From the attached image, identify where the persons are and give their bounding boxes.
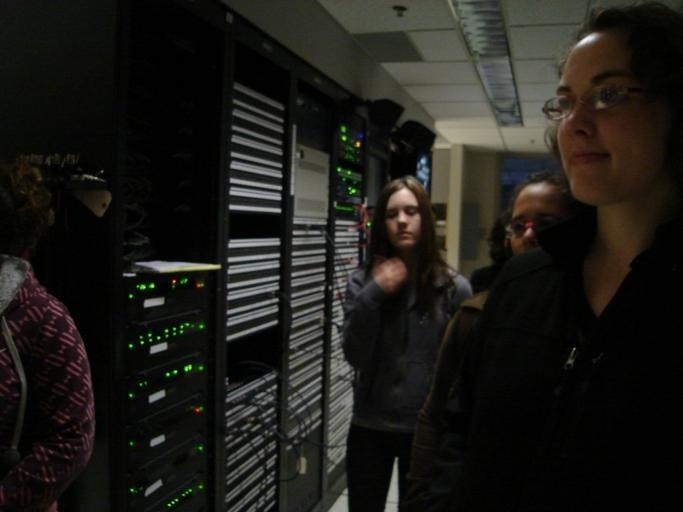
[0,153,96,510]
[470,205,513,294]
[427,0,682,512]
[341,174,474,512]
[399,168,569,511]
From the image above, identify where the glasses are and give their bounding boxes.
[496,220,545,240]
[541,82,646,122]
